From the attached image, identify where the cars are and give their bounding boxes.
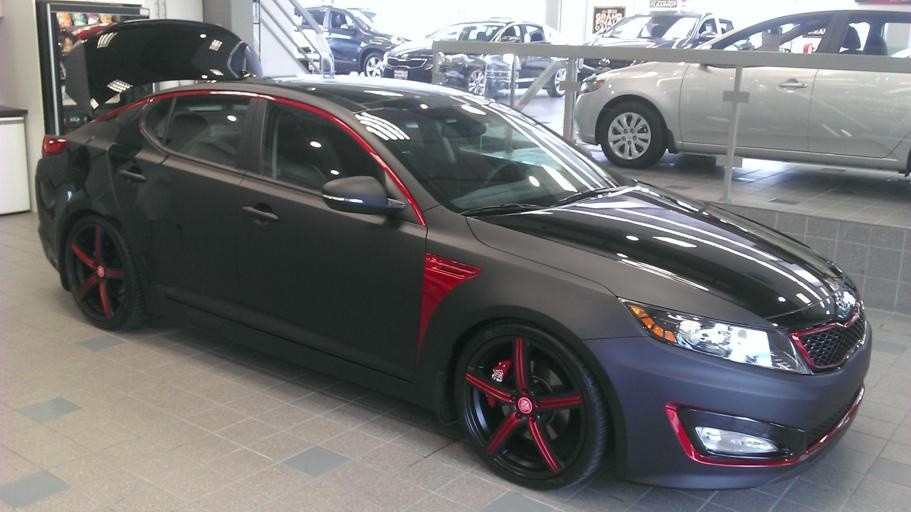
[574,3,911,177]
[575,8,734,84]
[378,18,570,98]
[30,19,874,494]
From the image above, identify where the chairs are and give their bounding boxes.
[168,113,236,167]
[840,27,862,56]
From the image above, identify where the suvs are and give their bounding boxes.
[292,5,413,78]
